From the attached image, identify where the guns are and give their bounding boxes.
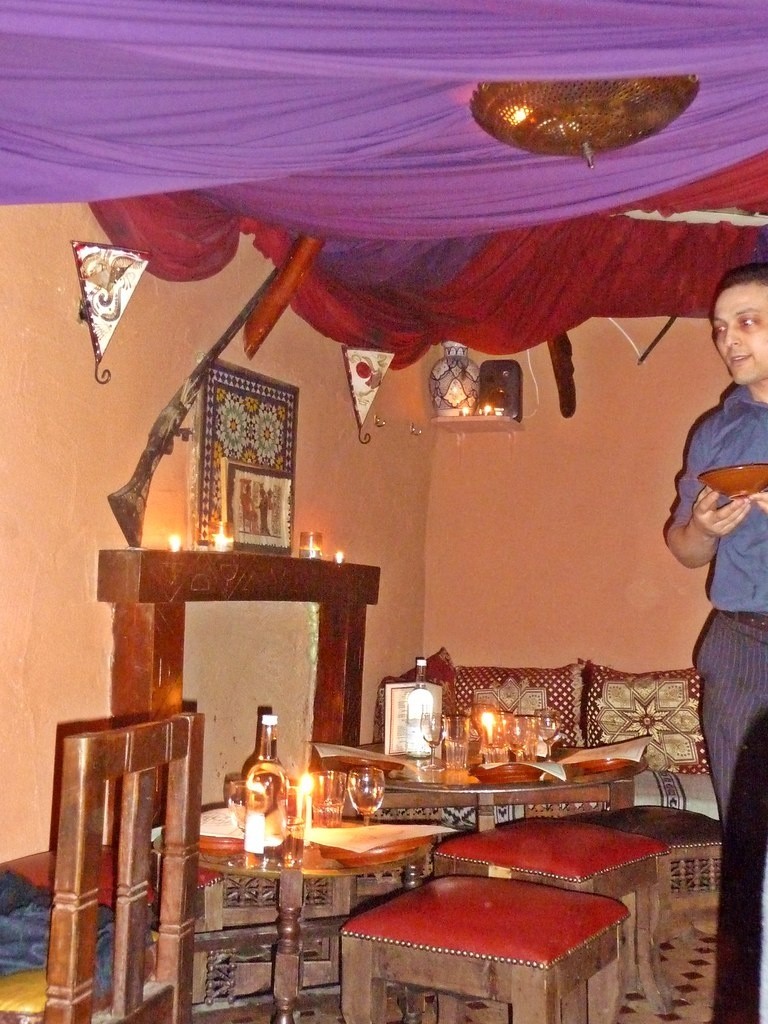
[109,261,280,548]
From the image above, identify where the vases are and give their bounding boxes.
[428,339,479,417]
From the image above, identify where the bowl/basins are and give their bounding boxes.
[198,835,243,858]
[320,835,433,869]
[698,463,768,500]
[336,756,405,780]
[469,765,545,783]
[569,756,637,775]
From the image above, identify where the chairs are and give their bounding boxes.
[1,711,206,1024]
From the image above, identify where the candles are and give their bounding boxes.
[298,771,315,847]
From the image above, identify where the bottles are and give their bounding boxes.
[407,658,436,758]
[429,341,480,417]
[244,714,289,855]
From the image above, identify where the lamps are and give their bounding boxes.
[466,74,701,169]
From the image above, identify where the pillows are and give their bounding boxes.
[450,658,589,752]
[583,661,710,776]
[372,645,457,741]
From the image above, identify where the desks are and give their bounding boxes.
[152,819,437,1024]
[334,747,646,836]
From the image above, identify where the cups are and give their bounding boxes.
[298,531,323,560]
[419,704,558,773]
[223,766,385,867]
[208,520,235,552]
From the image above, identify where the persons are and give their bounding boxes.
[663,260,768,826]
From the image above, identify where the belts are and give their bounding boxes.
[723,608,768,632]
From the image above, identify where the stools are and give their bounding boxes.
[433,817,671,1023]
[337,874,631,1024]
[585,804,724,996]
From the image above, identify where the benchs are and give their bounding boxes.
[375,772,723,909]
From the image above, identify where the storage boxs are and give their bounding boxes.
[384,680,444,755]
